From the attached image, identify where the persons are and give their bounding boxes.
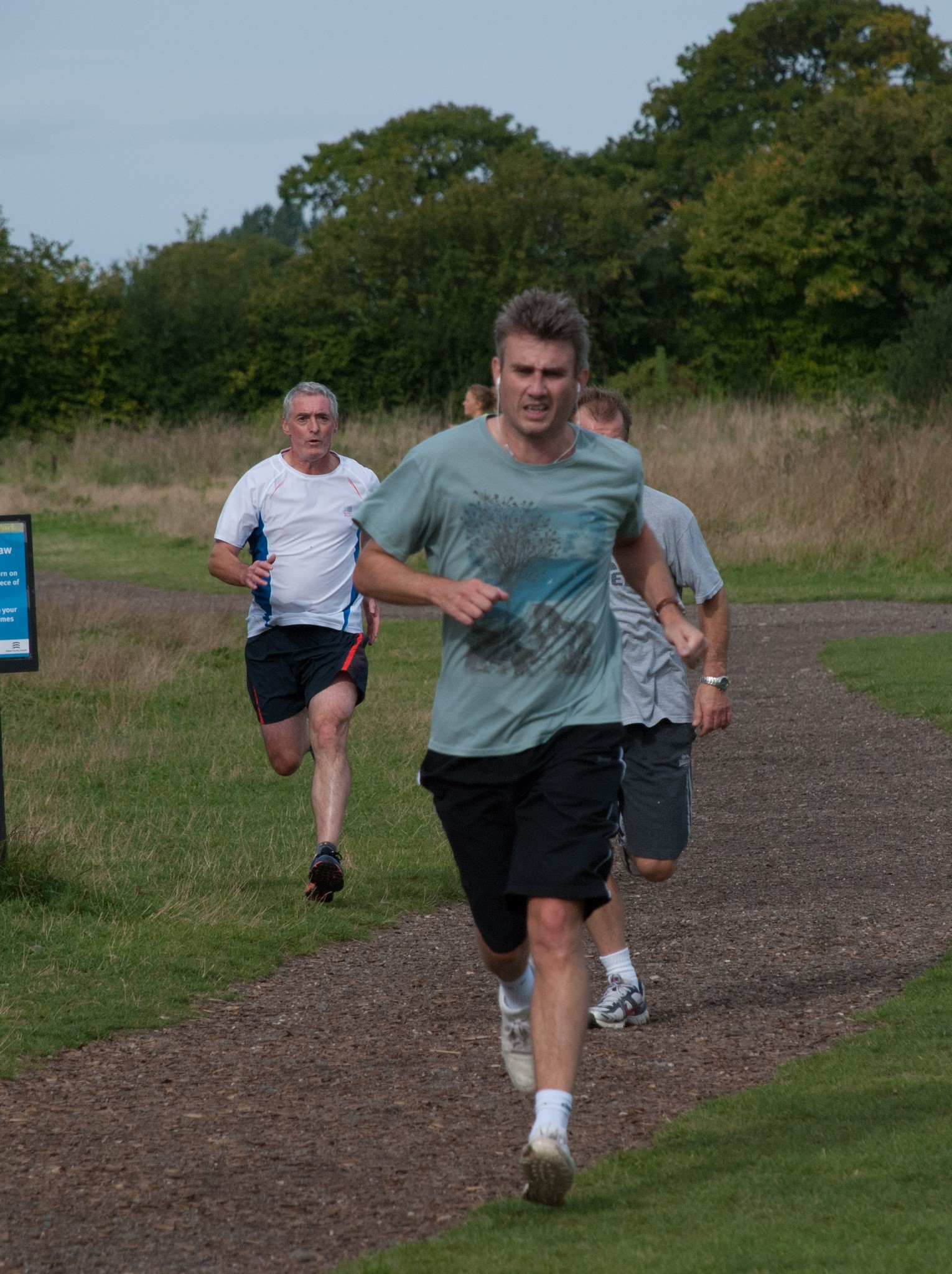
[569,391,732,1027]
[462,384,495,418]
[351,287,710,1207]
[207,382,381,901]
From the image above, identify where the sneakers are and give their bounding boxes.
[588,972,651,1028]
[304,846,345,903]
[519,1121,577,1208]
[499,957,538,1095]
[615,811,643,877]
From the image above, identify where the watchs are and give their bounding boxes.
[655,597,686,623]
[700,675,730,691]
